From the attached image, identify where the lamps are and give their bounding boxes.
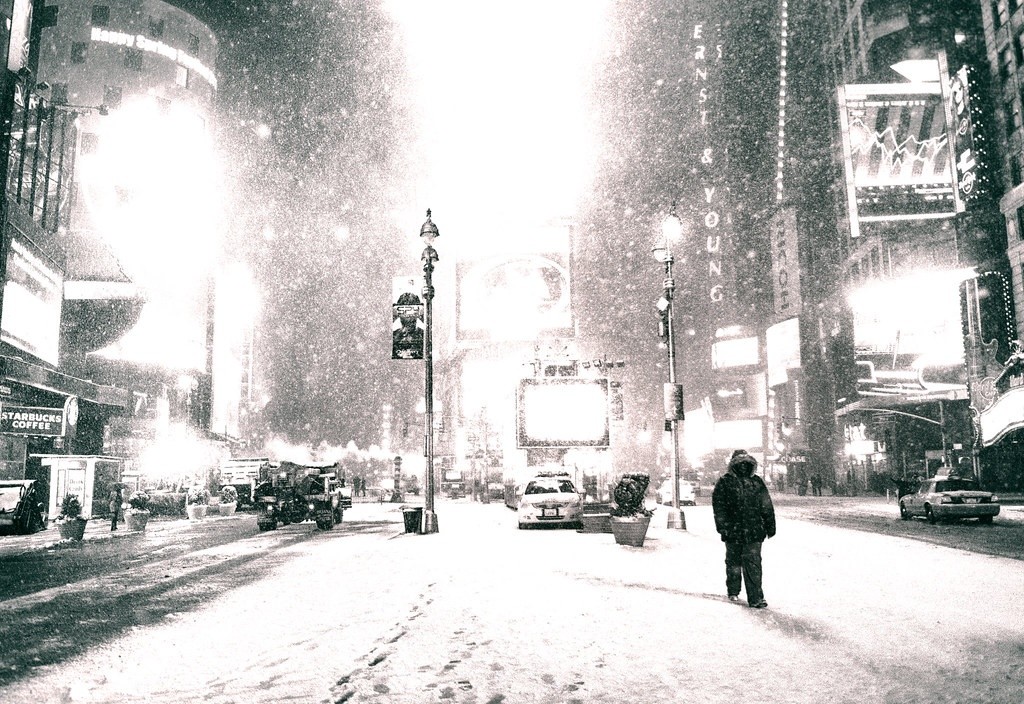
[37,81,50,91]
[18,66,32,77]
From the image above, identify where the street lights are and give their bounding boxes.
[846,401,949,467]
[649,198,693,530]
[418,208,440,532]
[779,412,849,496]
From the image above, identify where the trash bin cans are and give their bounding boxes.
[402,506,422,536]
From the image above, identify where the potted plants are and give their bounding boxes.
[607,473,657,546]
[218,486,238,516]
[52,492,88,540]
[187,490,210,520]
[124,491,150,530]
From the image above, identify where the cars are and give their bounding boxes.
[515,469,586,528]
[655,478,697,507]
[897,464,1002,524]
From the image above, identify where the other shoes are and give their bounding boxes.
[749,600,767,608]
[729,595,738,601]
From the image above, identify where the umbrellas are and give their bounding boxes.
[105,482,129,490]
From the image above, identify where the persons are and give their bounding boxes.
[353,475,366,498]
[109,496,118,531]
[116,489,125,522]
[810,473,823,497]
[712,449,777,610]
[341,478,345,488]
